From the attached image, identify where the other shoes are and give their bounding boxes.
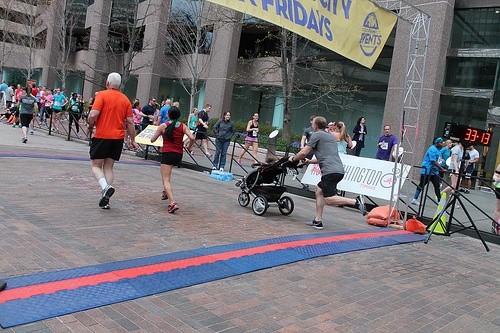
[464,190,470,194]
[30,131,34,135]
[204,153,213,158]
[220,168,224,171]
[237,162,243,166]
[458,188,465,192]
[188,150,194,155]
[0,118,22,128]
[21,137,27,143]
[450,203,460,208]
[411,200,422,206]
[212,166,216,170]
[492,225,500,235]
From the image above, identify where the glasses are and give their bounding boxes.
[152,100,155,102]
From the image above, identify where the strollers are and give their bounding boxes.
[235,156,308,216]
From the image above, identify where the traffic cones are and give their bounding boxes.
[426,191,448,234]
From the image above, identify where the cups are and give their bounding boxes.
[149,117,154,121]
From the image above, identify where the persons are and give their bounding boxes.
[0,80,100,134]
[87,73,138,209]
[352,118,368,156]
[212,111,235,171]
[488,164,500,235]
[186,108,198,155]
[297,114,353,154]
[289,116,366,229]
[150,107,194,214]
[124,96,180,155]
[17,87,39,143]
[376,125,398,161]
[238,112,261,168]
[190,104,214,157]
[413,137,480,210]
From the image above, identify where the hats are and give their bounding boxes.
[467,142,473,146]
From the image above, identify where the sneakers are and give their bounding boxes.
[306,218,324,229]
[167,202,179,213]
[101,198,110,209]
[98,184,115,207]
[355,194,367,216]
[161,190,168,200]
